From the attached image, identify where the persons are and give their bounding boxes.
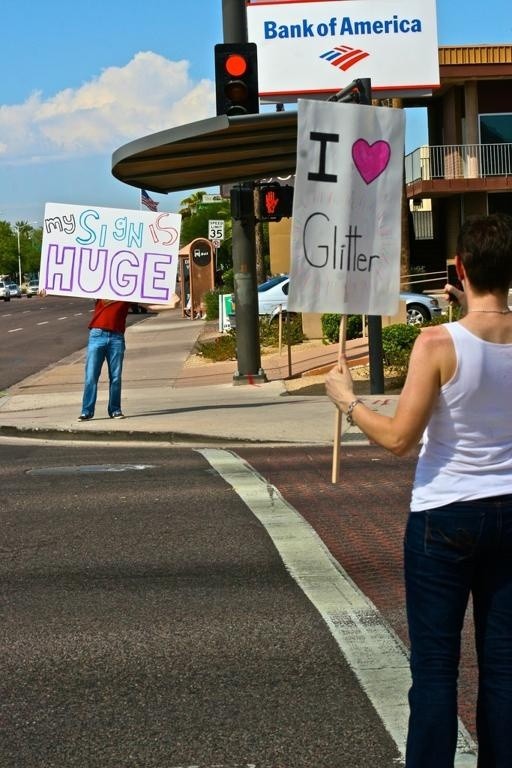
[324,215,512,768]
[38,289,180,422]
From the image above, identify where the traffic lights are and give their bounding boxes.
[259,186,293,217]
[229,186,255,220]
[213,42,260,117]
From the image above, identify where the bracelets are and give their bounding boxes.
[346,400,363,427]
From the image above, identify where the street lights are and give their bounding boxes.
[9,221,39,286]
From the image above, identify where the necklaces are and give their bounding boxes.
[474,307,510,315]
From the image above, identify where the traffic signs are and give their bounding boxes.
[208,219,224,241]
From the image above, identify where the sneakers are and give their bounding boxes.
[76,412,93,421]
[111,413,125,418]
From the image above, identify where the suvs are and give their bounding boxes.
[25,280,39,298]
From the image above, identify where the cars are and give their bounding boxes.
[0,280,22,302]
[256,271,444,331]
